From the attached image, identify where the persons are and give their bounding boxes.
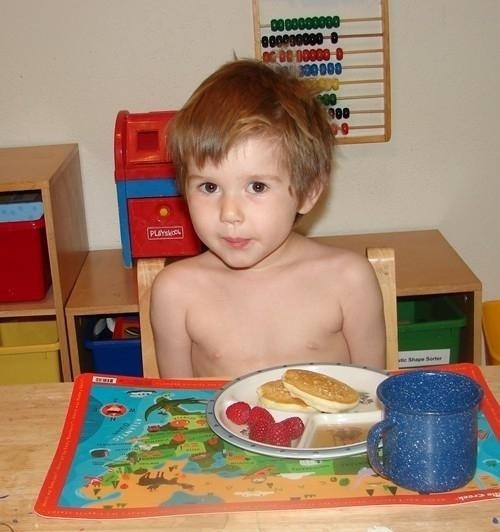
[150,60,387,378]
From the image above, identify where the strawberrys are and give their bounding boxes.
[279,417,305,440]
[249,420,271,443]
[248,406,275,427]
[225,401,251,425]
[267,423,291,447]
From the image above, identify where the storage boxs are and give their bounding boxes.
[86,315,142,379]
[1,200,50,305]
[1,318,65,384]
[397,296,468,369]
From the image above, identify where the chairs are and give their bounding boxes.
[138,248,399,379]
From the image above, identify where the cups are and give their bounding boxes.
[366,370,485,495]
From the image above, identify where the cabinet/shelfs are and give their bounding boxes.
[65,229,483,382]
[0,144,90,382]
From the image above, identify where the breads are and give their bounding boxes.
[259,369,361,413]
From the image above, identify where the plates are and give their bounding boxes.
[214,366,388,450]
[205,363,390,461]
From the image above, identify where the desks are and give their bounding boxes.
[0,366,499,532]
